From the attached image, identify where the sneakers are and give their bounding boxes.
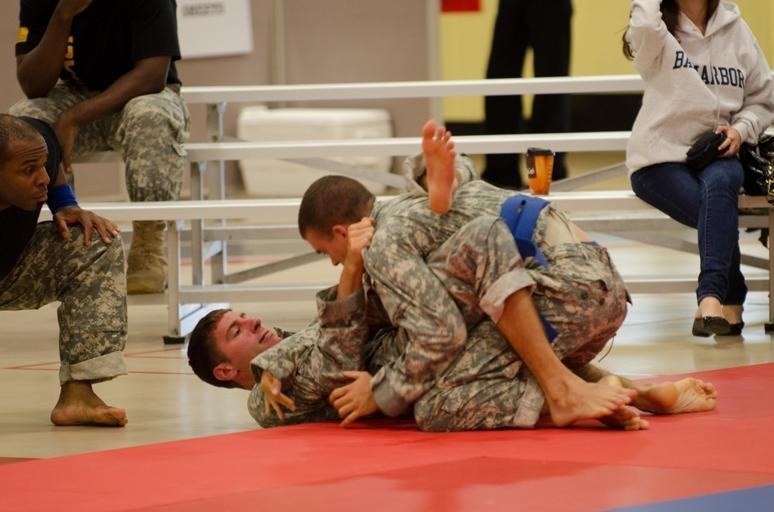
[126,229,169,294]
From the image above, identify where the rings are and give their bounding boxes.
[727,135,734,143]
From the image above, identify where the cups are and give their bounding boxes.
[524,147,555,197]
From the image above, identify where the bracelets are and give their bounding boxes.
[45,182,79,216]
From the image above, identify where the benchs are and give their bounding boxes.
[37,74,772,335]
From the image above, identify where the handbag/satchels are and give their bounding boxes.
[739,133,774,197]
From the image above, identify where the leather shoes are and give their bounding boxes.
[714,304,745,337]
[692,315,726,338]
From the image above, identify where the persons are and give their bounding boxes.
[621,0,773,340]
[0,110,133,429]
[184,115,643,429]
[294,171,720,434]
[1,0,192,299]
[473,0,575,194]
